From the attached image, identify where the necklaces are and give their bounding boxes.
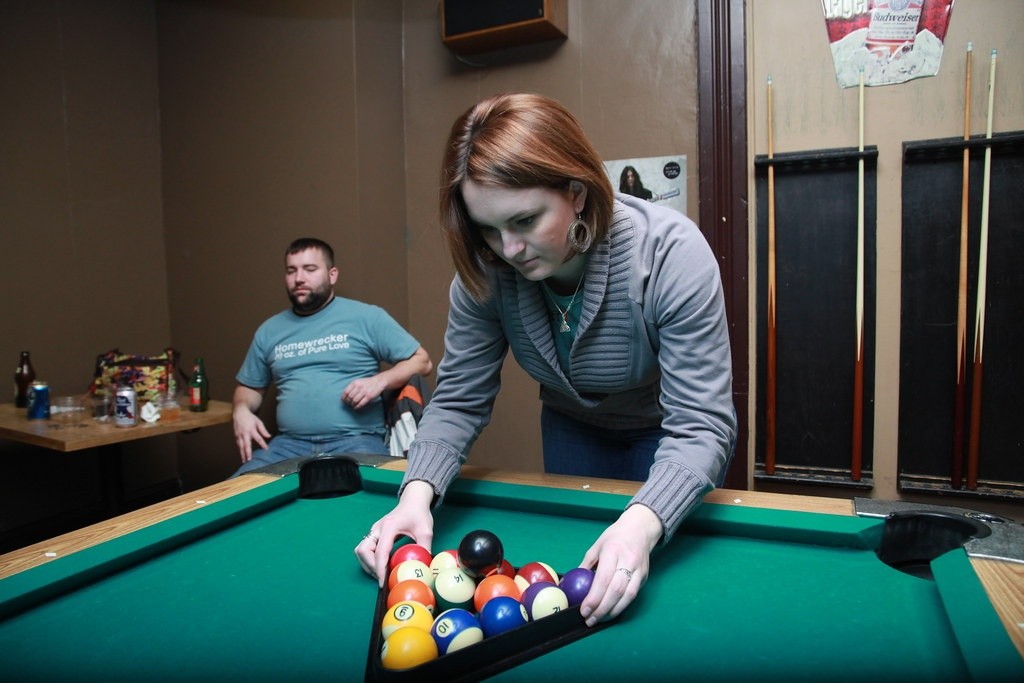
[542,270,584,333]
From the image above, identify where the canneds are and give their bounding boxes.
[114,386,138,427]
[26,381,50,421]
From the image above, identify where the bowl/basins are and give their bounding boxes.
[50,395,90,425]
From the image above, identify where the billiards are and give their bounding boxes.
[380,527,596,671]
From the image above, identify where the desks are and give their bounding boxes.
[0,450,1024,683]
[0,391,234,522]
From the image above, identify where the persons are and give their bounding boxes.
[354,93,737,629]
[226,238,434,481]
[619,166,652,200]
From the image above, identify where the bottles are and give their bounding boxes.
[189,358,206,411]
[14,351,36,408]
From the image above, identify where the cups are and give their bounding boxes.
[94,391,109,421]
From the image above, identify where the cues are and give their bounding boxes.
[947,40,1001,490]
[848,65,866,484]
[766,73,776,476]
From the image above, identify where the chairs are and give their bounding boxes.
[383,375,425,457]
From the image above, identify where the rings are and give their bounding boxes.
[363,530,378,543]
[616,568,631,578]
